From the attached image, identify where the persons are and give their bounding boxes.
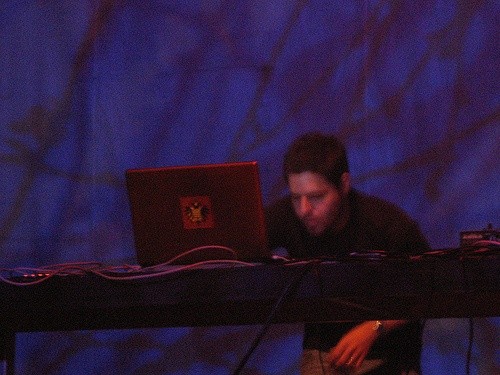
[254,133,432,375]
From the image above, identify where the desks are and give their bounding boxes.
[0,252,500,375]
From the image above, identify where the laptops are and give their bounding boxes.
[126,161,272,267]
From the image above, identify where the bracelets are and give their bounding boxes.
[376,320,383,338]
